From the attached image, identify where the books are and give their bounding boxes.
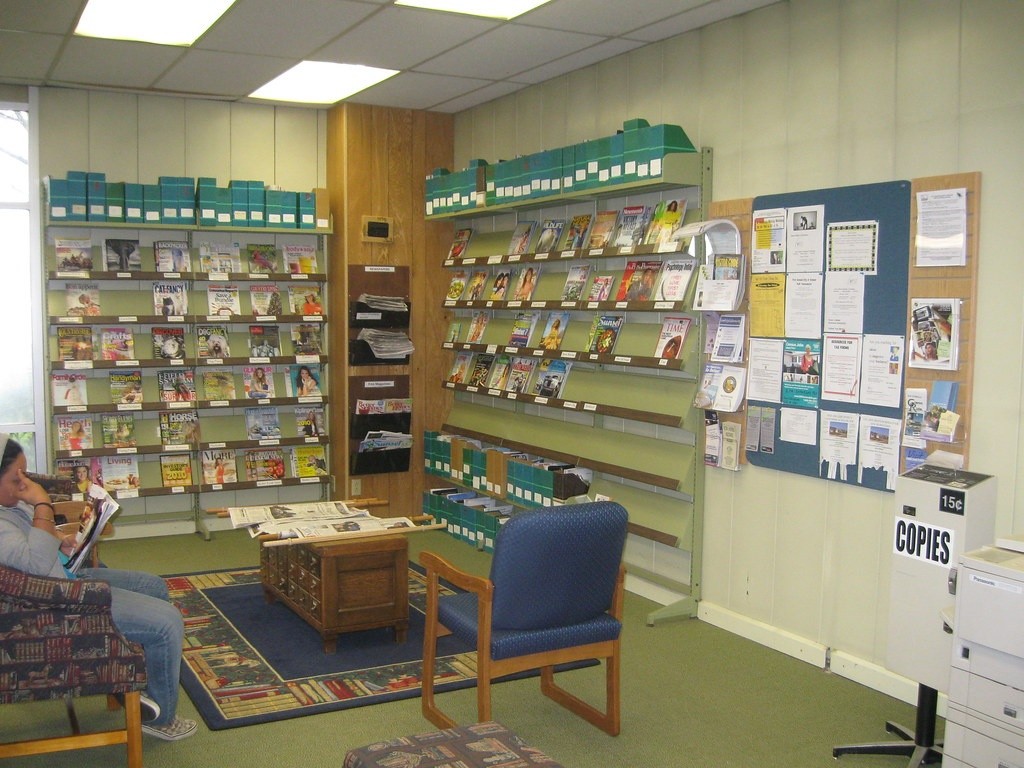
[64,484,120,572]
[52,200,697,494]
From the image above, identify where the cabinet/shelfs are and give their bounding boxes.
[418,145,716,627]
[935,543,1024,767]
[41,172,337,543]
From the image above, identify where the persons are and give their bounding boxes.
[307,455,325,470]
[215,458,224,483]
[471,311,485,342]
[496,273,509,297]
[72,466,89,493]
[517,268,533,300]
[802,344,815,373]
[172,377,190,402]
[598,279,608,302]
[0,432,198,740]
[128,474,138,485]
[69,422,91,450]
[926,344,937,361]
[251,250,274,272]
[543,319,562,351]
[295,366,322,396]
[496,363,510,389]
[249,368,270,399]
[303,293,322,316]
[494,273,504,293]
[450,362,466,383]
[573,224,586,247]
[472,274,486,300]
[657,201,680,242]
[252,337,280,357]
[303,411,318,437]
[801,216,809,230]
[628,268,652,300]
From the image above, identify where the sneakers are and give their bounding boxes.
[141,716,198,742]
[115,686,160,722]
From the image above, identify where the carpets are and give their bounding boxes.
[160,560,600,733]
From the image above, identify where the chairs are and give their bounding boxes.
[416,500,630,736]
[0,565,150,767]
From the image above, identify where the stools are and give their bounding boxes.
[343,721,564,768]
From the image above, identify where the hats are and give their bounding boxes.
[303,290,315,298]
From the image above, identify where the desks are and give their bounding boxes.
[259,531,407,656]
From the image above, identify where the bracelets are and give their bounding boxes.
[32,517,54,523]
[34,503,54,511]
